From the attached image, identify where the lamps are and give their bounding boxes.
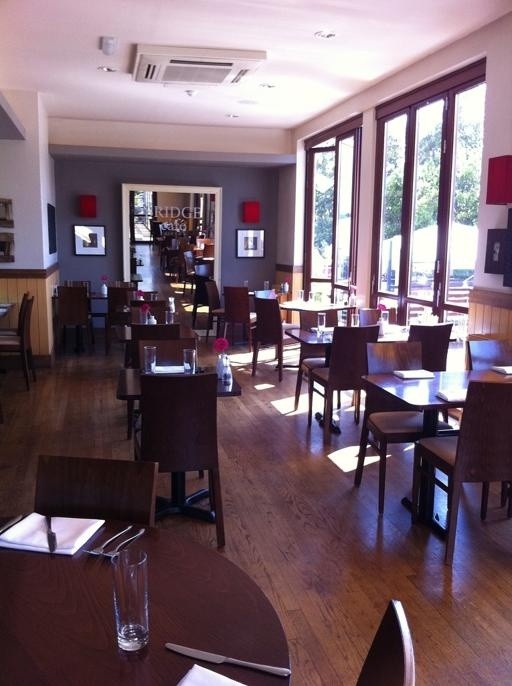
[486,155,512,205]
[79,195,96,217]
[242,201,260,223]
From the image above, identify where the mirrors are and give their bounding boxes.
[122,183,222,340]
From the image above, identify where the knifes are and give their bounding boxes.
[44,512,57,552]
[165,642,291,678]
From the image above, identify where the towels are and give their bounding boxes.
[0,513,105,555]
[176,664,248,686]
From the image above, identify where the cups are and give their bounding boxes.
[151,293,159,301]
[381,311,389,326]
[111,549,149,652]
[351,314,359,327]
[297,290,304,304]
[183,349,196,375]
[165,310,173,324]
[144,346,156,374]
[318,312,326,335]
[133,291,137,301]
[244,281,248,287]
[309,292,314,303]
[264,281,269,290]
[139,310,147,324]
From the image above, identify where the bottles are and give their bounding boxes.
[377,318,384,338]
[284,281,289,292]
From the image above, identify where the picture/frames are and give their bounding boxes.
[236,228,265,259]
[73,225,106,256]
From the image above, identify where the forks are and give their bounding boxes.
[83,525,133,555]
[99,528,146,557]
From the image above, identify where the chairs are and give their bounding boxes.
[356,599,416,686]
[149,219,214,329]
[34,452,160,527]
[0,291,37,390]
